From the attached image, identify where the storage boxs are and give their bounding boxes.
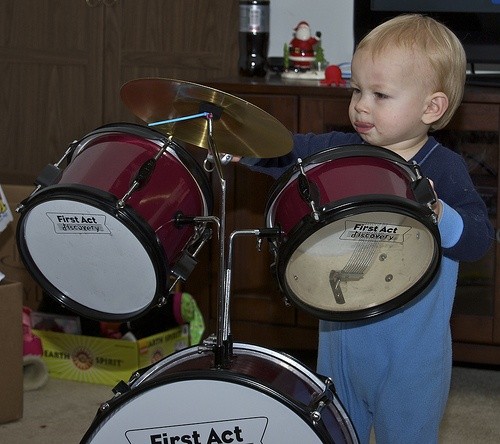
[31,324,188,387]
[0,184,36,281]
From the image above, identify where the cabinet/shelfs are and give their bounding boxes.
[186,77,500,367]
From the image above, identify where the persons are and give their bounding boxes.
[220,14,495,444]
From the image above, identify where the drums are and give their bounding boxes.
[264,143,443,323]
[15,122,215,324]
[79,342,360,444]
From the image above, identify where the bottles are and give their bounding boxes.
[238,0,271,79]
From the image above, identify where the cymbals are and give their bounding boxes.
[119,77,294,159]
